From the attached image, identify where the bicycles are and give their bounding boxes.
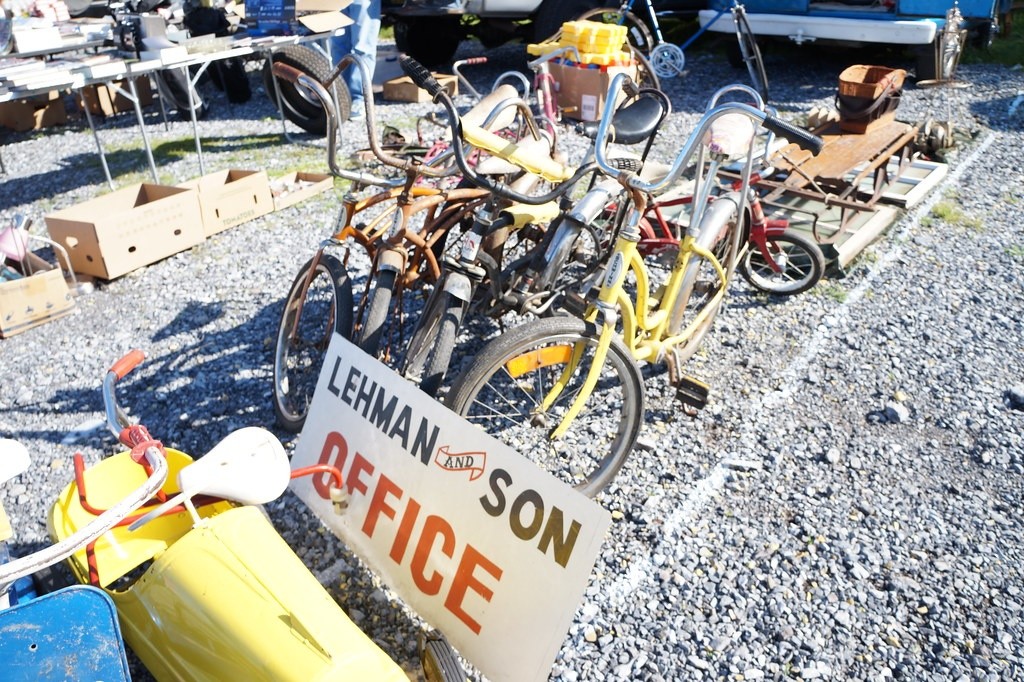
[546,0,773,105]
[266,43,828,494]
[68,0,206,122]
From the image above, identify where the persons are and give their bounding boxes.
[320,0,383,121]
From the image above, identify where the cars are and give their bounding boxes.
[697,0,1024,82]
[378,0,620,72]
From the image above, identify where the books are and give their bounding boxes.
[0,14,292,96]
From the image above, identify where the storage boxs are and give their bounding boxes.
[269,171,334,212]
[245,0,356,36]
[547,61,637,122]
[178,168,275,241]
[0,250,75,340]
[45,181,208,280]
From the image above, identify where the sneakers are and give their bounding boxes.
[348,93,367,121]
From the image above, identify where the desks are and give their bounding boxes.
[0,28,345,190]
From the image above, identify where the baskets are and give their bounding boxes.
[834,64,909,135]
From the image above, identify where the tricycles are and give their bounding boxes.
[2,351,475,682]
